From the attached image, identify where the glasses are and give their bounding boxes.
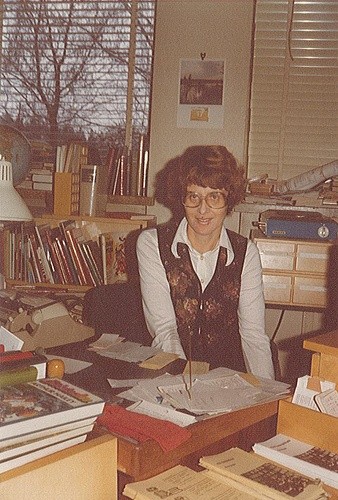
[181,191,228,209]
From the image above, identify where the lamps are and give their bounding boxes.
[0,157,33,222]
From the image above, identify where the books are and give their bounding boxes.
[247,161,338,206]
[0,377,106,474]
[31,143,80,192]
[291,375,338,416]
[0,221,126,286]
[246,211,337,306]
[106,145,149,196]
[123,434,338,499]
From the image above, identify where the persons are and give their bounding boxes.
[134,145,275,382]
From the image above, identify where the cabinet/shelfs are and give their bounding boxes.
[0,205,155,335]
[250,229,337,307]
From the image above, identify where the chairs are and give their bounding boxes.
[83,286,153,348]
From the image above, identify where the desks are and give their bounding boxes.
[304,331,338,390]
[0,336,338,500]
